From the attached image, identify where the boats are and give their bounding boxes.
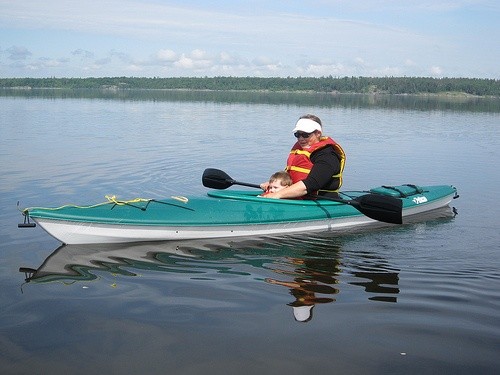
[15,184,459,246]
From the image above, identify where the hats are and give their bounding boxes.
[292,118,322,133]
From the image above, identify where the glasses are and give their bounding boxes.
[294,131,316,138]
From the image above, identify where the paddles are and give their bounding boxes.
[201,167,403,227]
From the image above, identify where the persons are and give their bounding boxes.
[260,114,345,199]
[260,171,293,196]
